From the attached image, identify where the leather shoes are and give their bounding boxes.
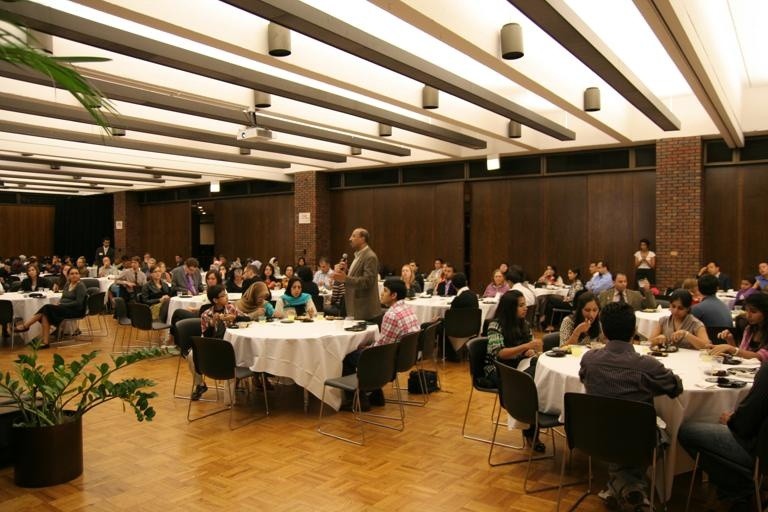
[10,324,29,333]
[367,390,385,407]
[33,343,49,349]
[534,443,544,452]
[2,332,9,337]
[340,398,369,412]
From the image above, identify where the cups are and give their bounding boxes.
[728,289,733,294]
[201,295,206,303]
[735,306,743,313]
[17,287,49,297]
[640,341,651,356]
[316,312,323,319]
[287,307,295,319]
[176,291,182,297]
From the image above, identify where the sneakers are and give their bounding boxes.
[191,382,207,400]
[262,379,275,391]
[252,378,264,391]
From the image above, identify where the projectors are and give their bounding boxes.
[236,128,273,142]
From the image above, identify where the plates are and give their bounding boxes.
[179,295,191,297]
[640,309,656,312]
[546,347,572,357]
[404,295,496,304]
[228,315,377,331]
[648,344,746,386]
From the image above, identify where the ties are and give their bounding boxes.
[135,272,137,284]
[617,292,625,305]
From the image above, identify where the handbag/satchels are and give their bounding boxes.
[408,369,439,394]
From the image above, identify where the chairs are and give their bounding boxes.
[82,279,100,297]
[462,337,550,449]
[1,300,23,353]
[435,308,482,369]
[319,341,405,446]
[385,321,438,404]
[684,419,767,510]
[488,360,592,493]
[352,329,426,420]
[112,298,153,354]
[187,335,269,432]
[535,294,575,330]
[172,317,235,401]
[4,276,22,292]
[126,303,172,360]
[556,392,668,512]
[57,291,109,341]
[542,333,561,352]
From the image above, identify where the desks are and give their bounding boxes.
[223,317,380,413]
[715,289,741,307]
[166,290,287,323]
[406,295,501,326]
[0,290,63,344]
[11,272,54,282]
[80,277,117,291]
[508,344,763,502]
[525,283,571,297]
[86,267,98,277]
[635,308,748,340]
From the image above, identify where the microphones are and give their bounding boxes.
[340,253,348,271]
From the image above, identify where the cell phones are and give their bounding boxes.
[732,381,744,387]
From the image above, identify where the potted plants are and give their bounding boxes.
[1,336,171,489]
